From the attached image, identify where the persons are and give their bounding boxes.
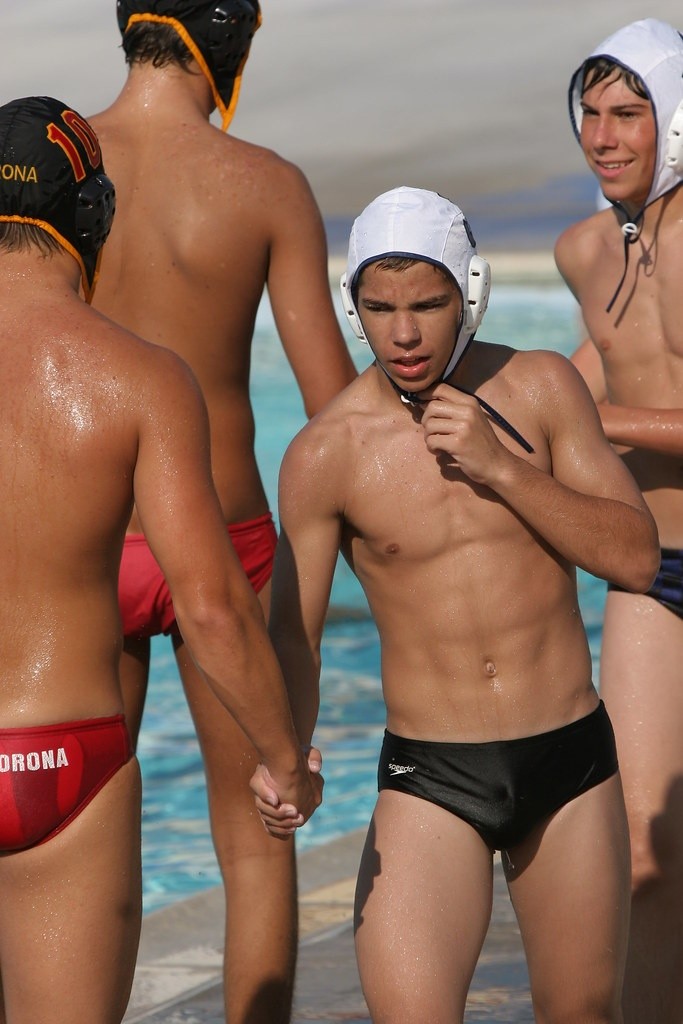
[86,1,359,1022]
[250,185,661,1024]
[553,17,683,1024]
[0,96,325,1024]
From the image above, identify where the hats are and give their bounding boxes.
[339,186,491,381]
[116,0,261,123]
[0,98,117,298]
[568,18,683,208]
